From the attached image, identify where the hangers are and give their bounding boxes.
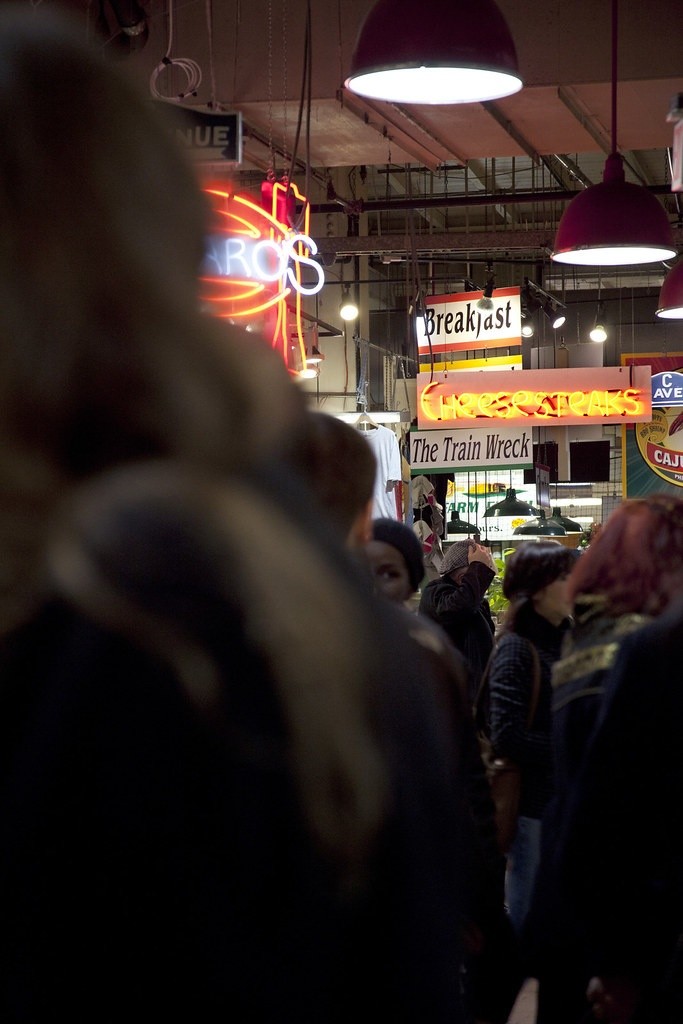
[344,396,395,439]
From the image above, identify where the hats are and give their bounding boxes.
[372,518,425,593]
[439,539,476,576]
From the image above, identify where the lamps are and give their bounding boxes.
[439,473,482,534]
[482,469,541,519]
[549,152,680,267]
[513,427,568,536]
[545,426,584,533]
[337,292,361,321]
[343,0,525,108]
[654,265,683,320]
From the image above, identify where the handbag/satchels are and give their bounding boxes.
[479,739,518,847]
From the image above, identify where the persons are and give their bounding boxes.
[3,0,466,1021]
[469,535,583,1024]
[366,517,430,619]
[420,540,496,702]
[514,492,683,1023]
[554,593,683,1024]
[266,415,523,1024]
